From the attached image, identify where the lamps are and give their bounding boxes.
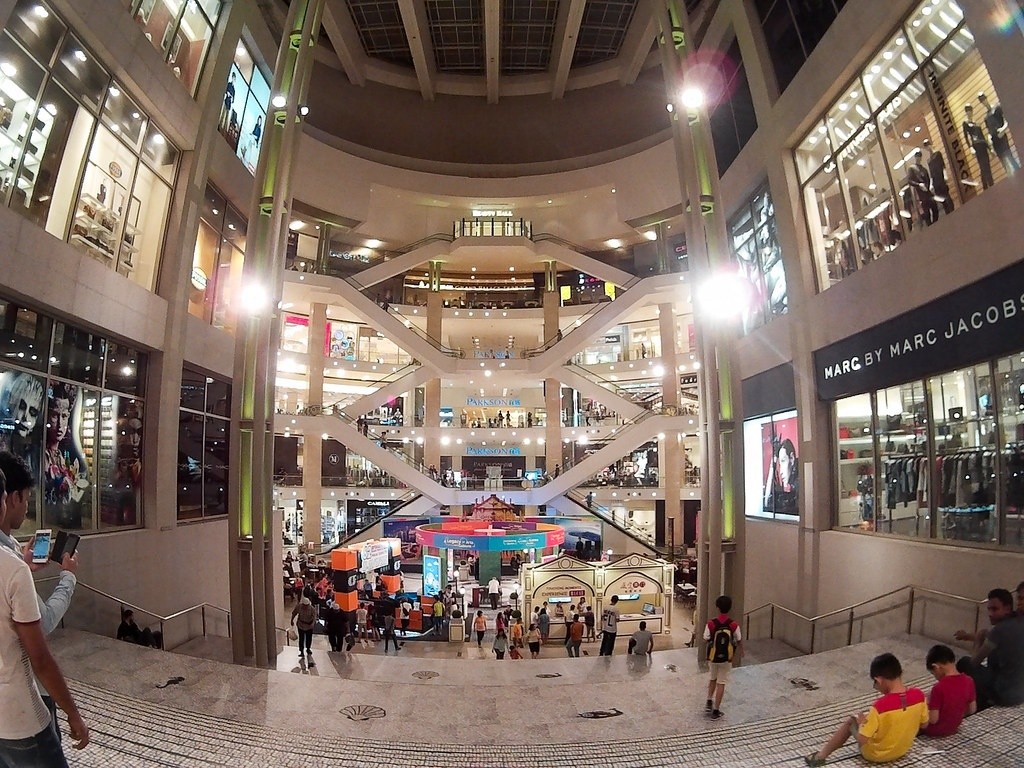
[666,102,675,113]
[298,104,311,117]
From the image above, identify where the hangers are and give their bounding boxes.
[886,442,1024,465]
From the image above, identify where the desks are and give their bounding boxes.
[395,607,423,630]
[472,587,504,608]
[548,617,593,638]
[616,613,661,635]
[309,569,320,584]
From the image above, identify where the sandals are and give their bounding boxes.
[804,751,826,768]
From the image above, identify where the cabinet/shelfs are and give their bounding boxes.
[71,193,144,269]
[79,391,119,489]
[0,68,56,209]
[841,432,970,466]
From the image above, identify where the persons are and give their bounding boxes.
[241,115,262,165]
[508,645,523,659]
[598,595,620,656]
[764,424,799,511]
[399,597,413,636]
[803,653,930,766]
[978,92,1021,175]
[525,623,543,659]
[0,452,90,768]
[920,644,977,736]
[267,260,700,671]
[628,620,654,655]
[221,72,237,132]
[908,152,939,226]
[473,611,487,648]
[1,373,144,489]
[291,598,316,656]
[115,610,162,647]
[565,614,584,657]
[952,581,1024,641]
[492,627,509,660]
[702,596,742,720]
[923,138,955,216]
[956,589,1024,706]
[963,103,994,191]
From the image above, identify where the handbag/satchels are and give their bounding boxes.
[286,626,298,641]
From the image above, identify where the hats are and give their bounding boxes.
[332,603,340,609]
[303,598,310,604]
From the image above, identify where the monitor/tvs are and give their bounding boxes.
[642,603,654,614]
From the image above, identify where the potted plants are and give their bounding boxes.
[452,611,463,623]
[510,610,521,624]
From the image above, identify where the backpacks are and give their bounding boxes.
[706,617,736,663]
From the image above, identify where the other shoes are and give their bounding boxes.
[298,653,305,657]
[306,649,312,654]
[705,699,724,720]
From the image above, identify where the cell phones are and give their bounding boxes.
[31,529,52,564]
[58,533,80,565]
[50,531,68,563]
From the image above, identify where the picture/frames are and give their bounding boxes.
[160,21,183,64]
[131,0,157,25]
